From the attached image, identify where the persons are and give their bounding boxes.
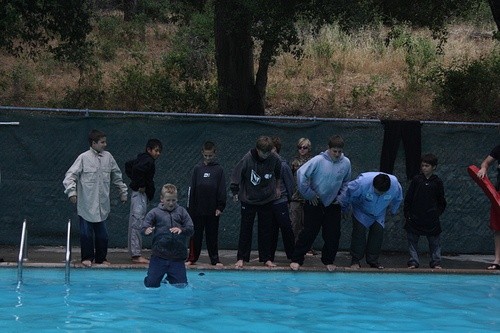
[342,172,403,269]
[271,136,293,262]
[230,136,282,267]
[403,155,447,269]
[63,129,128,267]
[477,145,500,269]
[288,138,315,244]
[290,135,352,272]
[185,141,226,266]
[141,184,194,288]
[125,137,162,263]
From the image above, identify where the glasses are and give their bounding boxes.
[297,146,308,149]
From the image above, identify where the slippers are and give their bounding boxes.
[487,264,500,270]
